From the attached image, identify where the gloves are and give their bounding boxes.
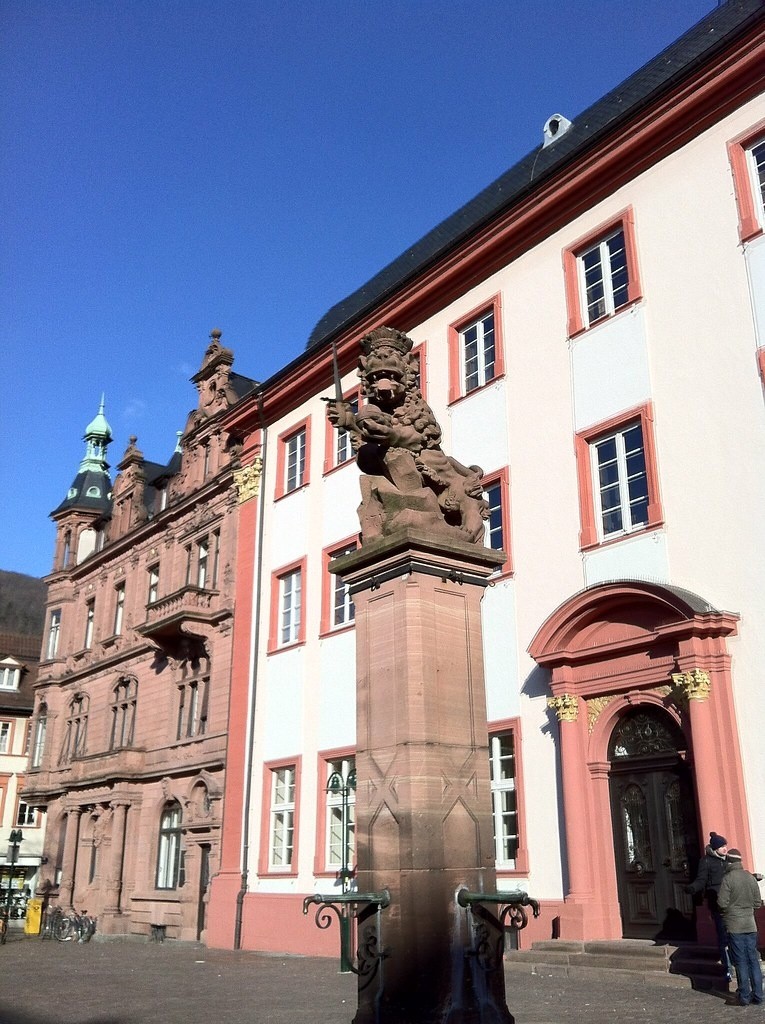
[752,874,762,881]
[678,885,695,894]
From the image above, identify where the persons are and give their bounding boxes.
[716,849,763,1005]
[678,832,763,983]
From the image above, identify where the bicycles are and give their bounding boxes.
[38,904,94,944]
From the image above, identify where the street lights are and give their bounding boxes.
[323,768,356,974]
[4,829,25,923]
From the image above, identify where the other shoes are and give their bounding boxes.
[725,971,732,982]
[751,999,760,1005]
[726,997,749,1006]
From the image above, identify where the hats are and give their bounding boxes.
[709,832,727,851]
[725,849,741,862]
